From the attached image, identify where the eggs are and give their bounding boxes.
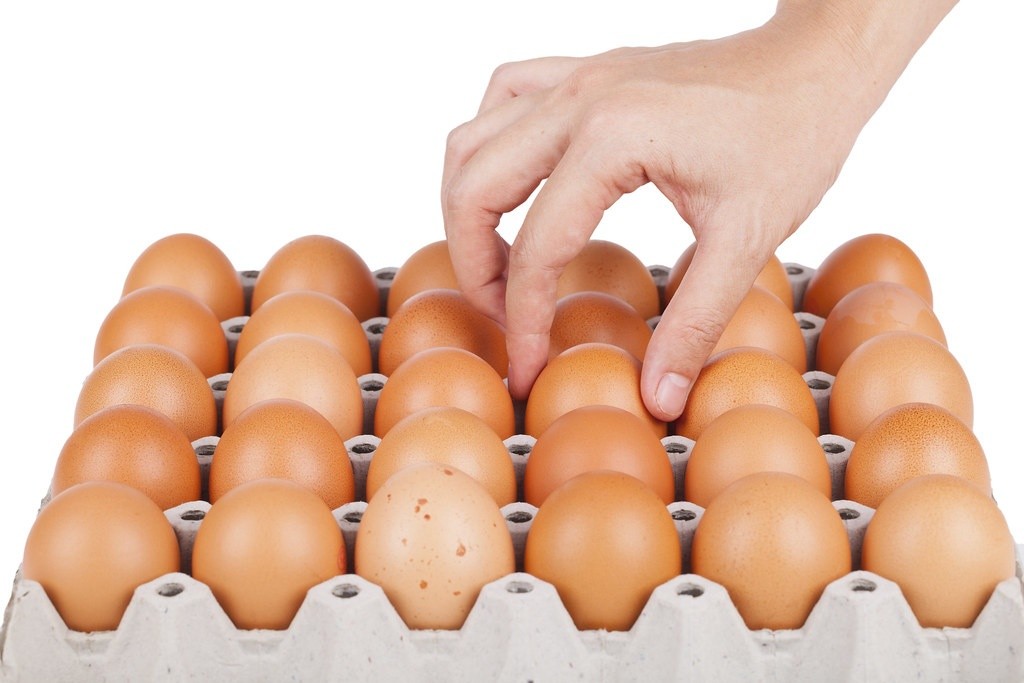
[24,225,1018,633]
